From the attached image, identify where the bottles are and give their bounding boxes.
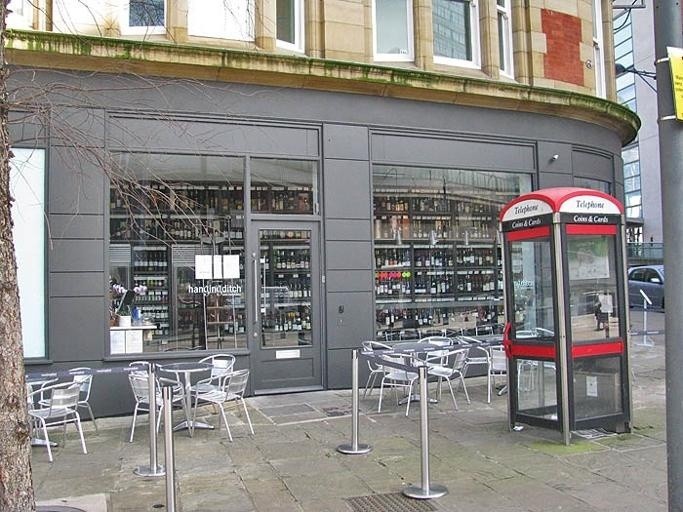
[130,243,309,270]
[374,270,502,296]
[375,305,523,332]
[133,276,309,301]
[107,186,311,214]
[140,305,310,335]
[110,218,311,238]
[372,196,499,214]
[373,248,502,268]
[371,216,501,243]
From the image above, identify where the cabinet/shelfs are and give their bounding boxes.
[374,193,524,341]
[109,183,311,348]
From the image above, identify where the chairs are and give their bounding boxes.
[26,366,98,461]
[362,332,533,417]
[127,353,254,443]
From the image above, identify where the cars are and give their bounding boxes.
[627,265,664,311]
